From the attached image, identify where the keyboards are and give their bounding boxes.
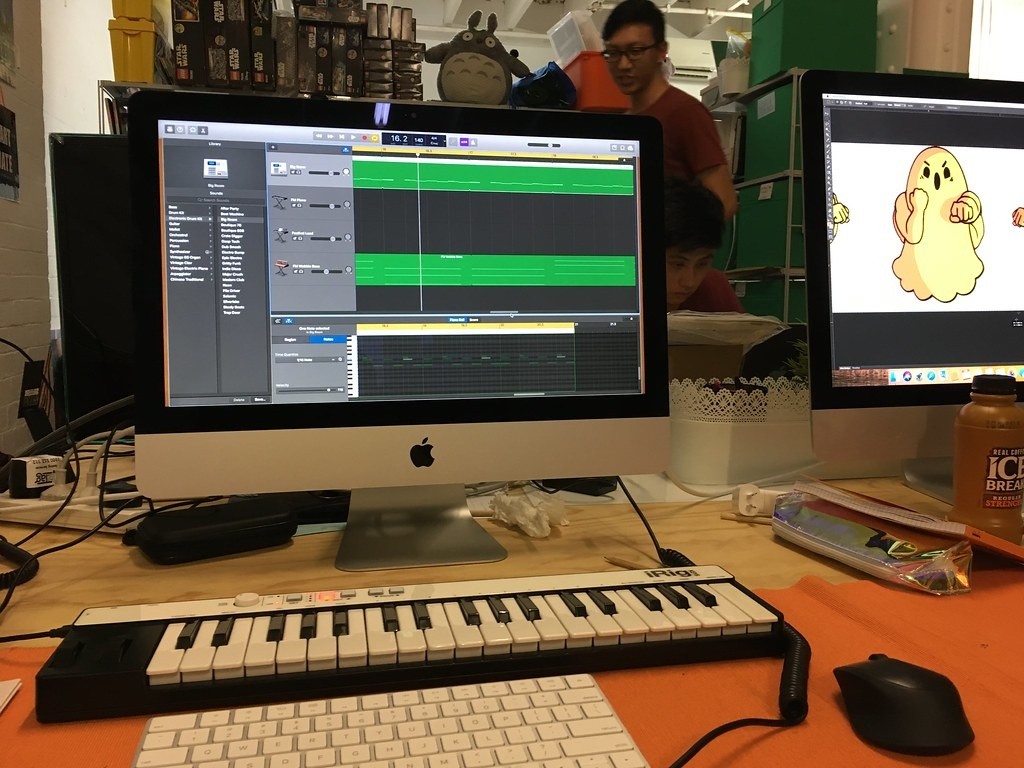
[131,673,650,768]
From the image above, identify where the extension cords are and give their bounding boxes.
[0,490,159,534]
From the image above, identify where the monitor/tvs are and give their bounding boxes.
[798,69,1024,505]
[50,89,672,572]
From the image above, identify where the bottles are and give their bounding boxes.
[953,375,1024,544]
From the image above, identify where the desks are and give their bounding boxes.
[0,424,1024,768]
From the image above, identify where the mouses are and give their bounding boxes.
[833,654,975,757]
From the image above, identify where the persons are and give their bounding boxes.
[663,183,740,316]
[603,0,736,222]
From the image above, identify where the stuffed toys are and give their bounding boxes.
[424,10,531,104]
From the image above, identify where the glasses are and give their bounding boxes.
[599,39,663,64]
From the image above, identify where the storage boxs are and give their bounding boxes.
[701,0,879,333]
[108,0,427,102]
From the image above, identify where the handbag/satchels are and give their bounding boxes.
[511,60,578,109]
